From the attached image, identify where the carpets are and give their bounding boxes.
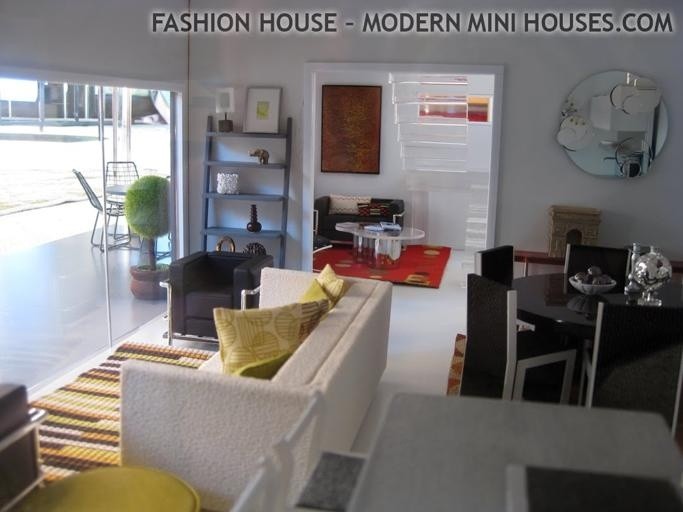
[446,331,466,395]
[313,239,450,289]
[28,341,213,468]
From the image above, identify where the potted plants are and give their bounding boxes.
[124,175,172,302]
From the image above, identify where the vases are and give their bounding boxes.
[246,203,262,232]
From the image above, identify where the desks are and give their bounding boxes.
[105,186,132,242]
[25,464,197,511]
[512,246,564,279]
[351,391,682,512]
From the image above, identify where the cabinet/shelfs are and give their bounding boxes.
[200,115,293,273]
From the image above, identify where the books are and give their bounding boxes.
[357,220,378,230]
[363,226,384,233]
[380,222,401,231]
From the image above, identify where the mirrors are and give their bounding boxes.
[556,67,669,181]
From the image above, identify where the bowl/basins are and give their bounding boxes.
[564,293,608,312]
[568,274,617,294]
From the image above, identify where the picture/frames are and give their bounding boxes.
[319,84,383,175]
[417,94,490,128]
[241,86,282,135]
[213,87,237,117]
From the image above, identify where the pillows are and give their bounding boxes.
[227,352,293,387]
[327,195,371,215]
[212,301,331,370]
[358,203,390,216]
[297,280,335,315]
[297,267,344,312]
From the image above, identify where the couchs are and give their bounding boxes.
[121,266,394,509]
[312,194,405,251]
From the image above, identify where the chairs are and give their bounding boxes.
[157,250,273,348]
[71,167,131,252]
[227,455,287,511]
[100,160,140,239]
[270,389,363,511]
[0,385,46,508]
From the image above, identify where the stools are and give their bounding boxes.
[313,236,332,257]
